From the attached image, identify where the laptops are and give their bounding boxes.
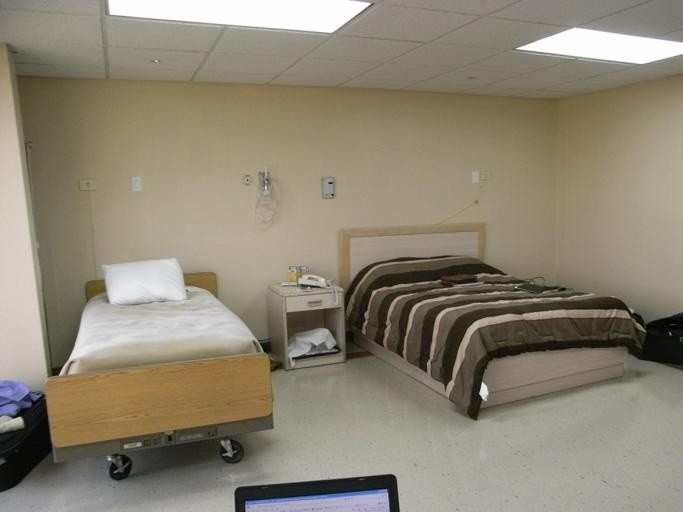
[234,474,400,512]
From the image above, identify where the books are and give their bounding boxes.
[440,274,483,287]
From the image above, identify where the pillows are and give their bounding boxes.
[99,256,189,308]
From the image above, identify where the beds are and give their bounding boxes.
[337,222,650,422]
[43,267,278,490]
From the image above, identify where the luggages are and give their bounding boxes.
[645,312,683,365]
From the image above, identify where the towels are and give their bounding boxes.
[285,328,335,367]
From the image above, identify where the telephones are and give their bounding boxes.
[297,274,332,289]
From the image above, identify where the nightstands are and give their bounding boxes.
[265,276,347,370]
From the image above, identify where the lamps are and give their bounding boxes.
[252,168,284,230]
